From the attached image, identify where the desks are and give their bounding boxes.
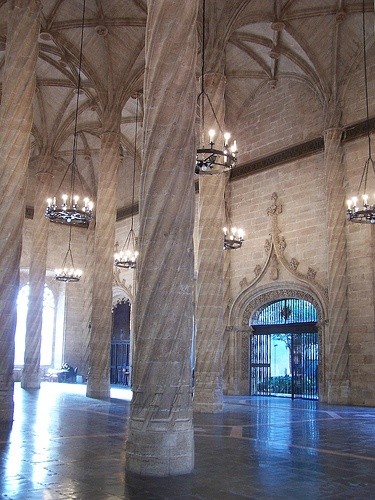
[52,369,70,383]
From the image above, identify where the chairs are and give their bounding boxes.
[71,367,78,382]
[68,368,77,383]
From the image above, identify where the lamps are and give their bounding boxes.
[345,0,375,226]
[45,1,96,229]
[195,1,239,175]
[223,226,245,250]
[54,133,82,284]
[112,89,141,270]
